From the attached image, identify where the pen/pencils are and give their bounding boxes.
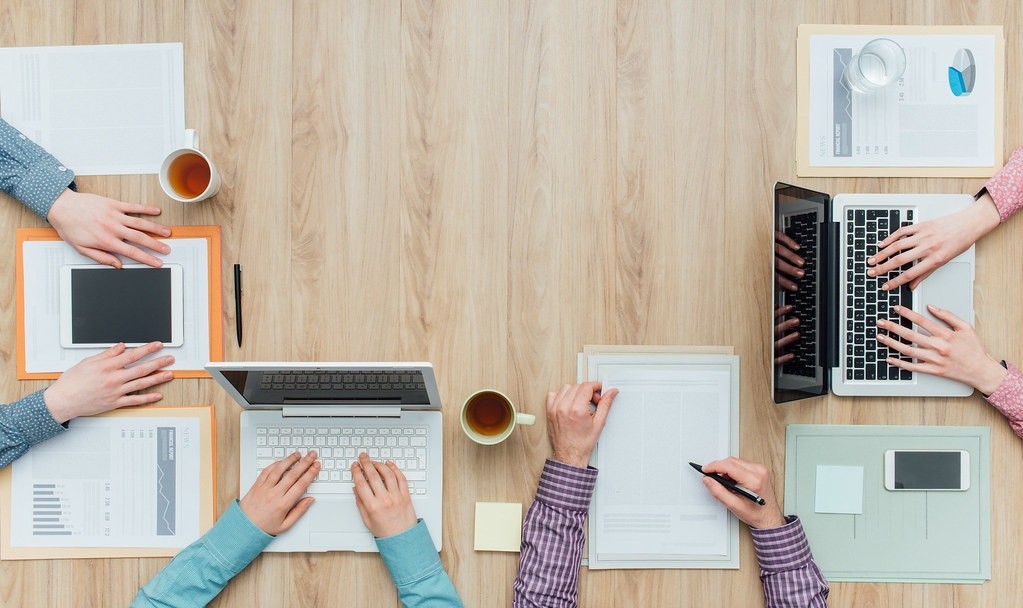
[688,460,767,506]
[234,263,244,348]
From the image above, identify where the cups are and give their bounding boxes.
[844,38,906,93]
[460,390,536,446]
[158,129,222,203]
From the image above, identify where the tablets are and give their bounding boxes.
[58,264,183,349]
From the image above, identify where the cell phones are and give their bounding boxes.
[884,450,970,491]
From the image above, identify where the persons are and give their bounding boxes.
[775,230,805,364]
[510,380,829,608]
[130,449,466,608]
[0,117,175,468]
[866,146,1023,441]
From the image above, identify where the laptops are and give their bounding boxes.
[769,181,976,404]
[203,360,444,559]
